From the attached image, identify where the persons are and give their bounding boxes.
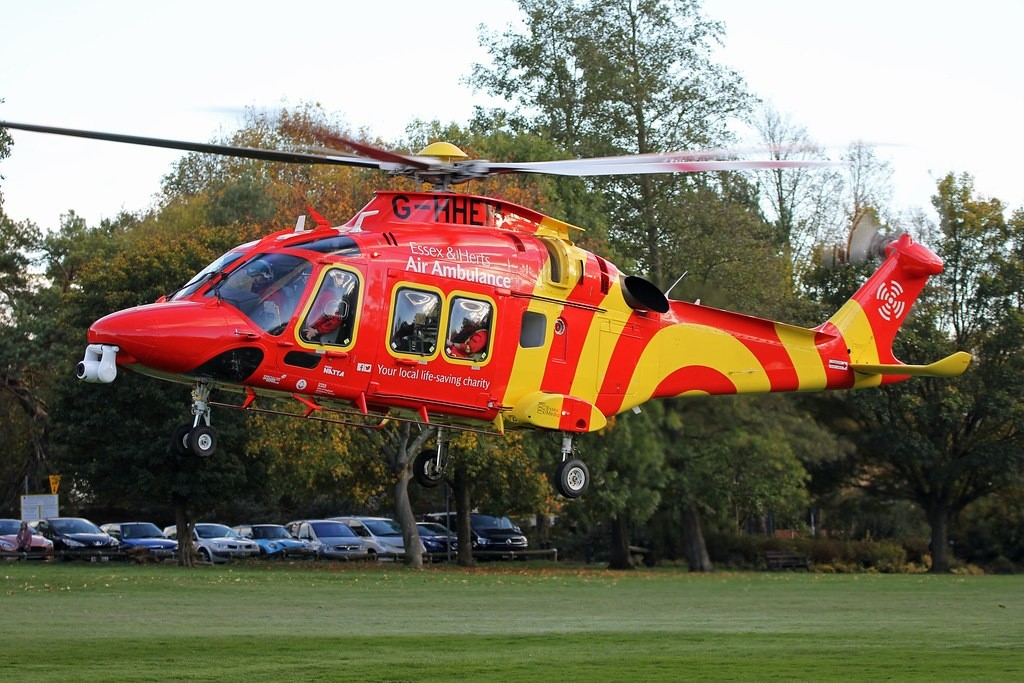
[246,259,284,327]
[447,305,488,359]
[295,268,342,341]
[15,521,32,560]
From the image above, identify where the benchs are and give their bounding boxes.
[764,550,810,572]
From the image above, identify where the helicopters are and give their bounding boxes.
[0,116,973,500]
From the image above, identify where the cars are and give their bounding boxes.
[27,517,119,561]
[285,520,369,563]
[325,517,427,563]
[232,523,312,560]
[99,522,178,562]
[162,523,260,563]
[415,522,459,562]
[0,518,55,562]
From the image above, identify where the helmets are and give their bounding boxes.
[300,267,312,275]
[246,259,273,282]
[469,312,487,327]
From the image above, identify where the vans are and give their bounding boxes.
[425,513,528,562]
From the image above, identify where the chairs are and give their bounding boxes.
[276,270,296,324]
[321,284,346,343]
